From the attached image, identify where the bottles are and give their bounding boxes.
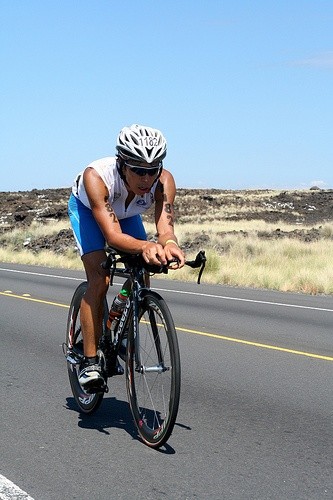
[107,289,128,331]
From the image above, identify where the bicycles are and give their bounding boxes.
[64,249,207,447]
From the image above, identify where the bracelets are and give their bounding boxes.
[165,239,178,245]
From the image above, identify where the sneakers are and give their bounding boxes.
[77,355,104,388]
[115,330,137,355]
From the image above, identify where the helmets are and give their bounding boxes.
[114,123,168,164]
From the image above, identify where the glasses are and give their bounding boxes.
[123,161,162,177]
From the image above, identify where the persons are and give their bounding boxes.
[67,124,185,387]
